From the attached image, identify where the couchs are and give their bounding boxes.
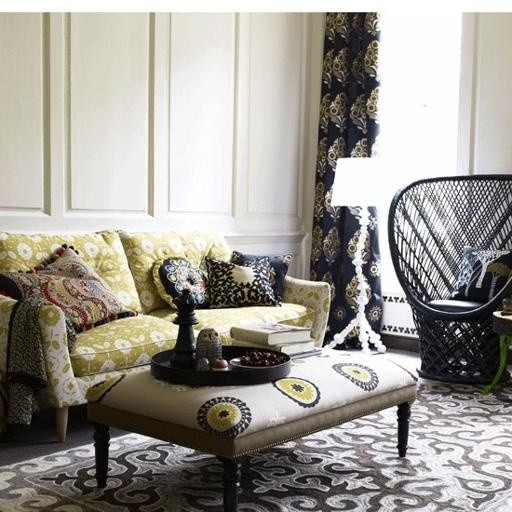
[0,228,333,441]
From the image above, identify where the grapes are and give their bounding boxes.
[241,352,286,366]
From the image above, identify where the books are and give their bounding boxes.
[230,339,323,359]
[229,319,312,345]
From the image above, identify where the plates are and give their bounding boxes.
[229,356,277,368]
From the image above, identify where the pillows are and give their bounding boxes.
[149,251,294,310]
[450,244,509,306]
[0,242,142,336]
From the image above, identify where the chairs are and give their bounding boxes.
[384,173,512,386]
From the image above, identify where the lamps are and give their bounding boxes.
[322,154,395,360]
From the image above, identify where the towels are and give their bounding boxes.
[5,296,60,428]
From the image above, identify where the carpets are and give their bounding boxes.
[0,377,512,507]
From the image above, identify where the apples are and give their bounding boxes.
[198,358,210,369]
[215,359,228,368]
